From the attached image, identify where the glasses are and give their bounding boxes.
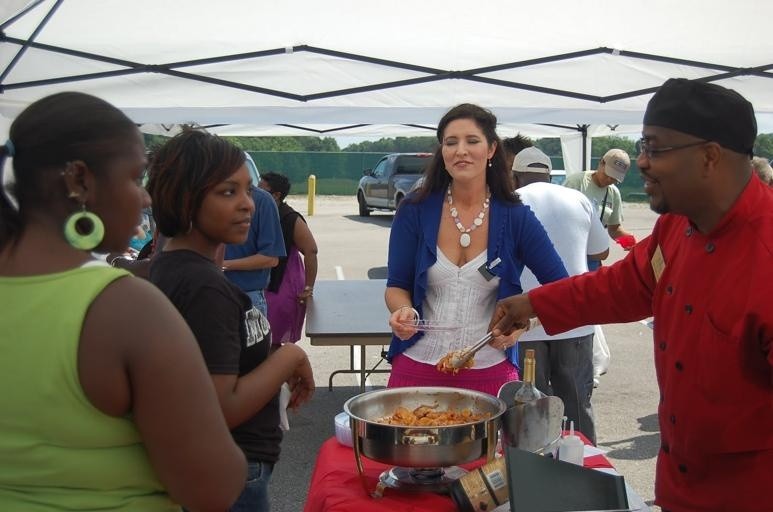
[634,136,713,159]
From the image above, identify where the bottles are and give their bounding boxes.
[515,349,540,405]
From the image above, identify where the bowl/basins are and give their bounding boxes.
[342,386,506,467]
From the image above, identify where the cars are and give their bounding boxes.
[357,153,435,217]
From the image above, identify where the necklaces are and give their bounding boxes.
[446,182,492,248]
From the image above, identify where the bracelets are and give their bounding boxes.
[303,286,314,290]
[393,307,421,329]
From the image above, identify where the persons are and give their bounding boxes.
[562,148,640,272]
[255,172,316,358]
[499,135,534,194]
[488,78,773,506]
[106,224,225,278]
[510,147,612,448]
[751,155,773,186]
[219,182,285,317]
[386,104,571,395]
[1,92,249,512]
[148,130,316,512]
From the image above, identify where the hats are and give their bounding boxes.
[643,78,758,161]
[511,145,552,174]
[602,148,631,184]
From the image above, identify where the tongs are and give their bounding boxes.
[441,327,499,371]
